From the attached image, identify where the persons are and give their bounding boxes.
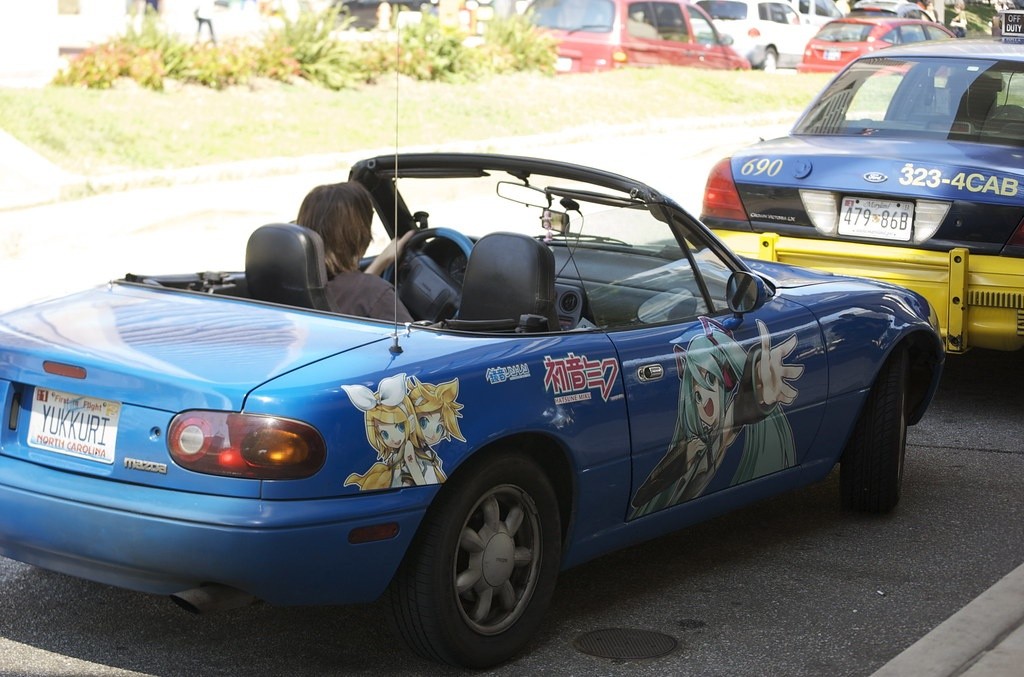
[987,1,1004,36]
[296,181,416,323]
[921,0,936,38]
[949,0,967,37]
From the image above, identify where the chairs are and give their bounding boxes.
[944,69,998,123]
[453,231,563,335]
[244,222,341,314]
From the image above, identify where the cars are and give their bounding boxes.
[692,1,934,72]
[510,1,750,80]
[0,149,949,667]
[796,18,955,78]
[703,39,1024,365]
